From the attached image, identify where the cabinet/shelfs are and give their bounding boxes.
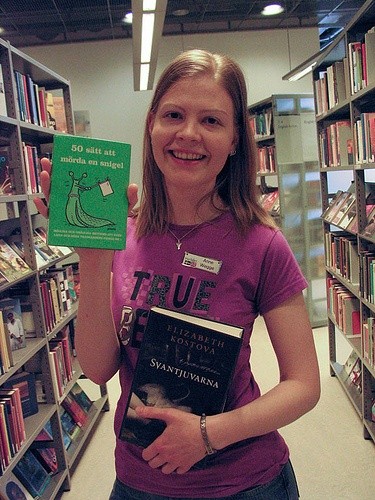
[247,0,375,444]
[0,38,109,500]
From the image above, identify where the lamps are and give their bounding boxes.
[132,0,168,91]
[282,51,323,81]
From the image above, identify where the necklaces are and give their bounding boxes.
[164,206,223,249]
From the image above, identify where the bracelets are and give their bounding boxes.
[200,413,217,454]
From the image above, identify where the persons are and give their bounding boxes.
[118,303,246,471]
[0,142,53,195]
[34,49,320,500]
[6,312,24,350]
[6,481,27,500]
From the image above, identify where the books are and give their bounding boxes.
[362,317,375,372]
[0,64,7,117]
[0,320,76,477]
[248,105,280,217]
[0,382,92,500]
[319,113,375,168]
[344,349,364,384]
[320,180,375,243]
[0,261,79,375]
[14,72,56,130]
[315,26,375,117]
[0,227,73,286]
[45,133,132,251]
[325,231,375,305]
[327,278,366,335]
